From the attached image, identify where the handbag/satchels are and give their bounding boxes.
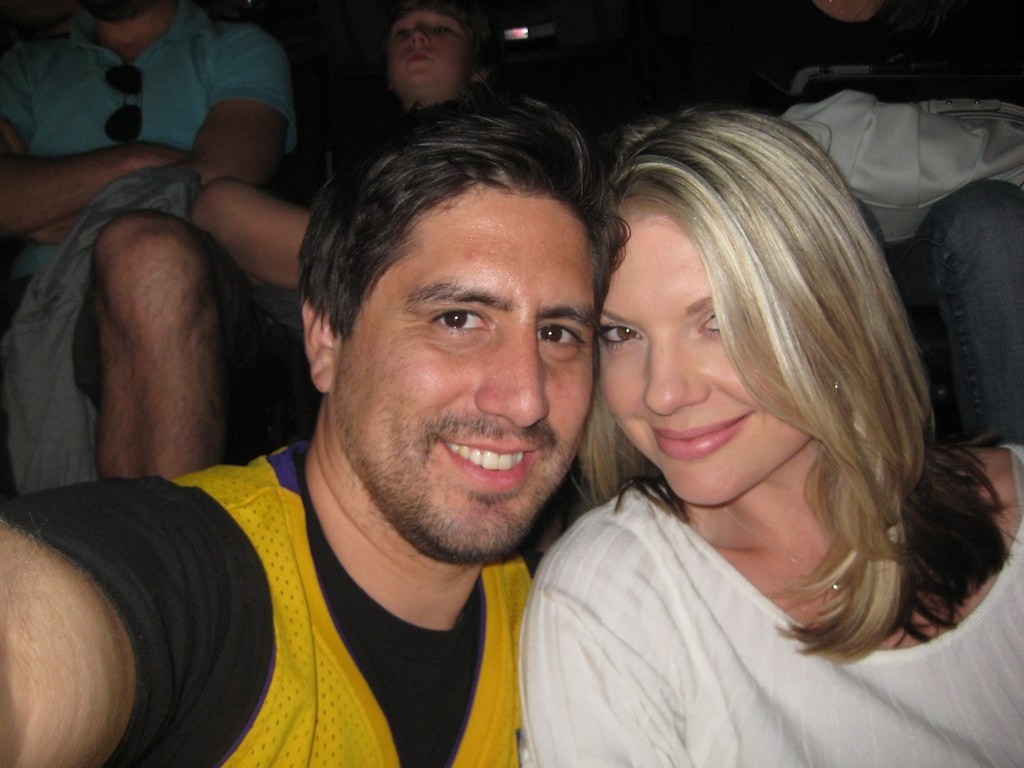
[782,91,1024,243]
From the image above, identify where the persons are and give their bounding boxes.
[0,1,1023,768]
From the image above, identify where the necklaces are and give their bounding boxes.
[726,508,845,591]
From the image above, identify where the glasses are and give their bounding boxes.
[104,64,144,144]
